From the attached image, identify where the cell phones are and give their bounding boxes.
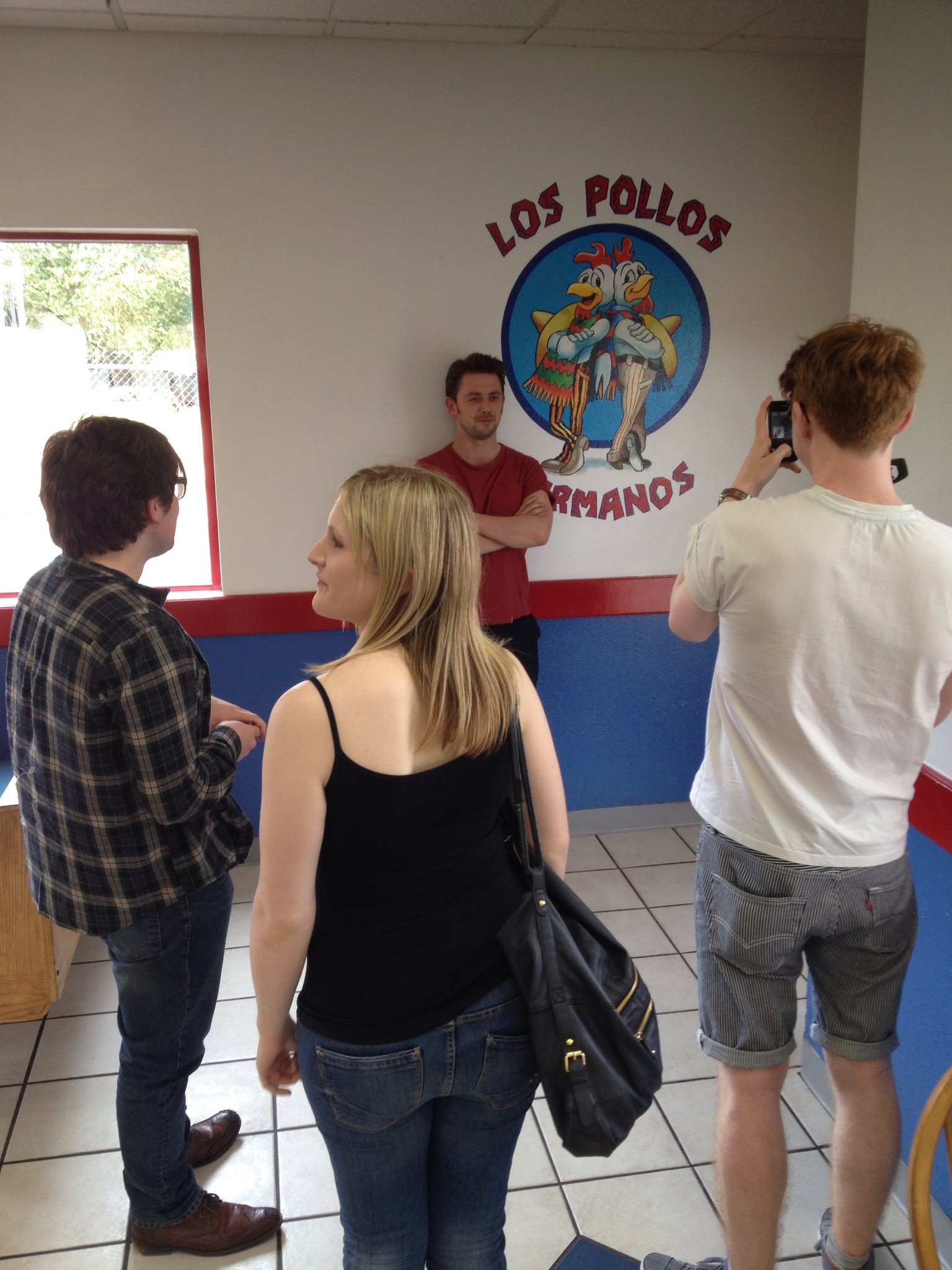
[768,400,797,462]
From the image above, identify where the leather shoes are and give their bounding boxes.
[186,1109,241,1169]
[132,1191,283,1257]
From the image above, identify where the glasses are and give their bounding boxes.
[173,464,187,499]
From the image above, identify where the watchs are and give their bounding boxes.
[718,487,751,504]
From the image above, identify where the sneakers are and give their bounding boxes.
[814,1208,876,1270]
[640,1253,728,1270]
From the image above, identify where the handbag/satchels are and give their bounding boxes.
[506,718,662,1159]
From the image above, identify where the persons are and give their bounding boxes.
[668,319,952,1270]
[415,353,556,698]
[774,433,778,438]
[5,415,283,1257]
[252,466,570,1270]
